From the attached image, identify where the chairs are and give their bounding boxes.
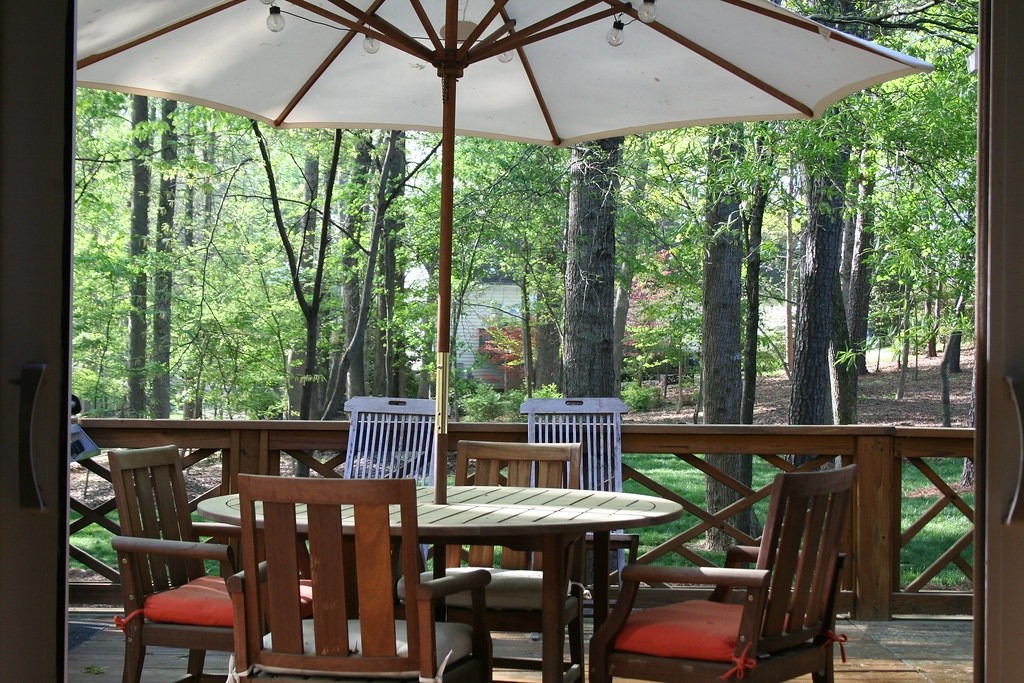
[106,445,271,683]
[519,398,629,590]
[343,396,452,563]
[227,473,493,683]
[397,440,586,683]
[589,463,857,683]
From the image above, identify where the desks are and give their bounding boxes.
[196,485,684,635]
